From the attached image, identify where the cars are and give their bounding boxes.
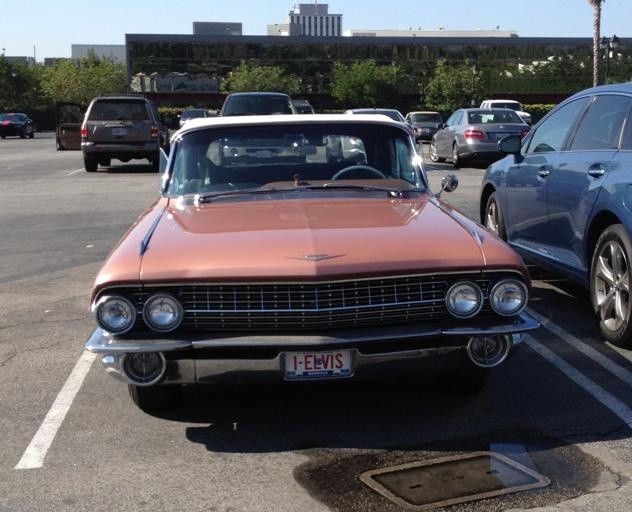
[180,109,207,125]
[218,92,309,163]
[291,98,314,116]
[326,107,417,168]
[478,79,632,348]
[79,112,545,416]
[430,108,532,166]
[0,111,35,139]
[476,100,532,125]
[406,111,445,142]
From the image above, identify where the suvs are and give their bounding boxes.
[55,93,172,176]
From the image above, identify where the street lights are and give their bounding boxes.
[470,64,481,107]
[211,71,226,110]
[599,34,621,85]
